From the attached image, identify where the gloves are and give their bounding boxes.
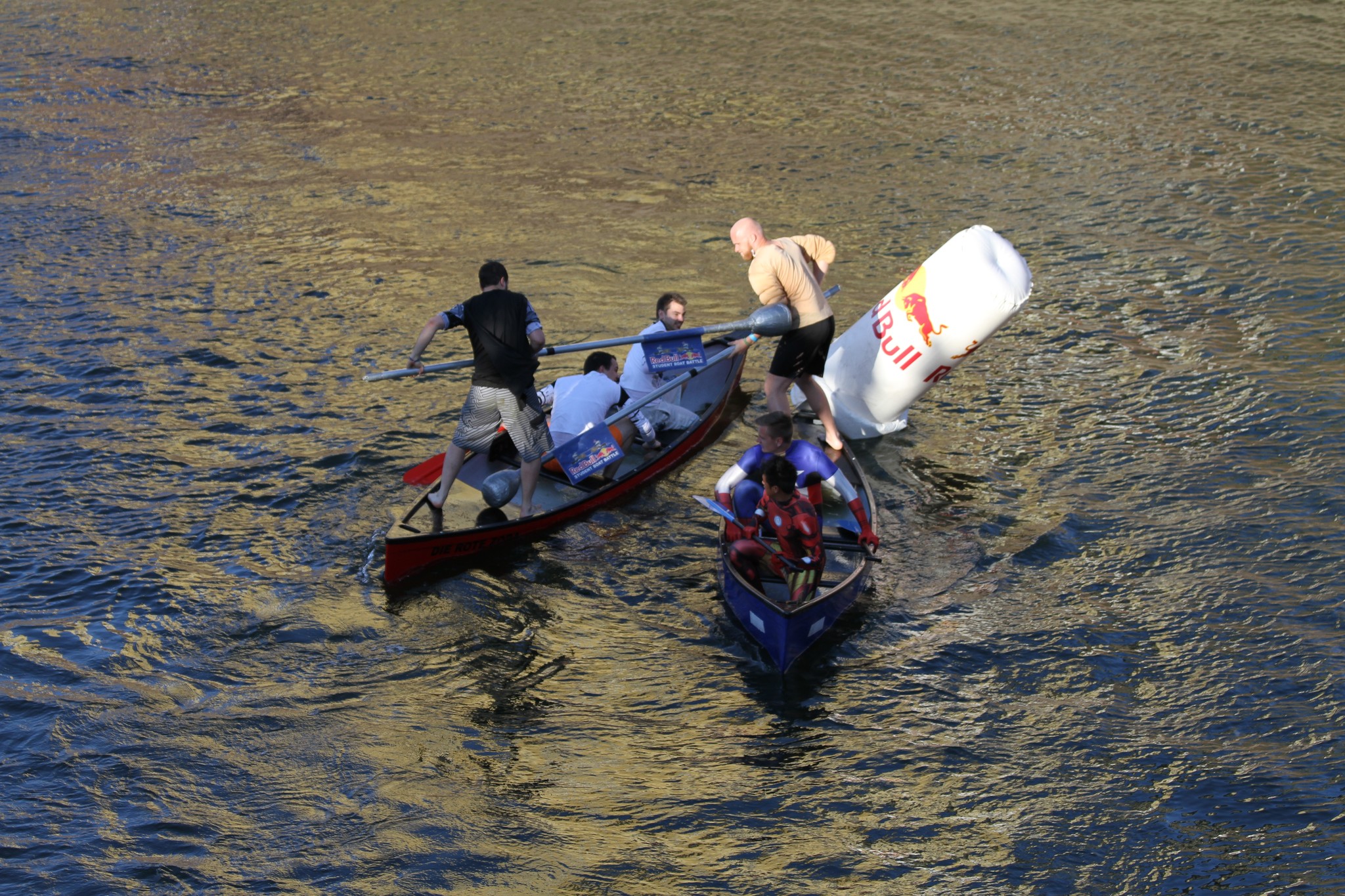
[714,490,741,541]
[771,551,796,574]
[742,518,759,539]
[849,497,879,554]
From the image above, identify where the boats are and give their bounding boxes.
[719,401,880,677]
[383,327,756,586]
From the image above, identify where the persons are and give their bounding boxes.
[618,292,701,430]
[500,352,663,481]
[724,217,844,450]
[714,411,880,556]
[724,455,826,605]
[408,260,553,517]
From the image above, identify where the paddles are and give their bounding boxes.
[402,426,505,486]
[692,494,804,573]
[837,519,883,563]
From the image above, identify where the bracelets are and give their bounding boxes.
[749,333,758,342]
[744,337,752,346]
[409,357,420,363]
